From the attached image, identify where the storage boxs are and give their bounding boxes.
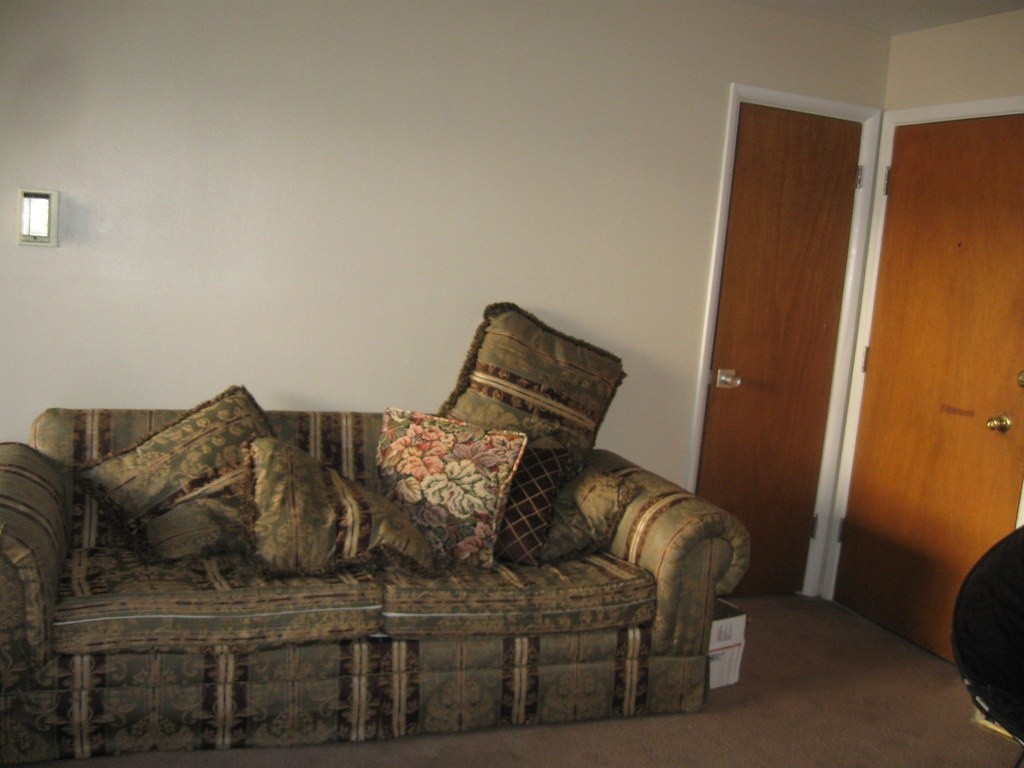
[708,598,746,689]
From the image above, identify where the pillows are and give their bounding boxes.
[244,434,448,579]
[436,305,624,450]
[496,449,568,562]
[72,386,277,567]
[542,467,638,558]
[371,408,527,573]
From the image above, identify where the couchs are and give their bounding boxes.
[0,408,752,768]
